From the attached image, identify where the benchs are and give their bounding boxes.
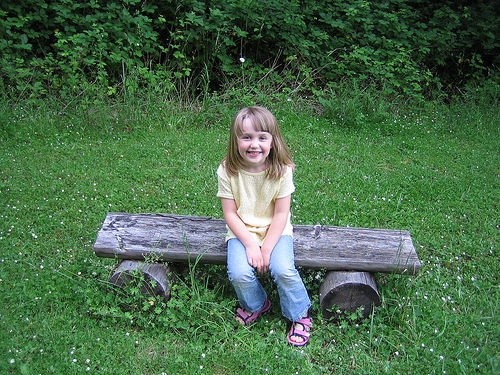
[92,211,421,327]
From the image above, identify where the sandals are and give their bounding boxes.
[287,316,313,346]
[234,298,271,325]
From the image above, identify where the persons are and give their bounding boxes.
[215,106,312,347]
